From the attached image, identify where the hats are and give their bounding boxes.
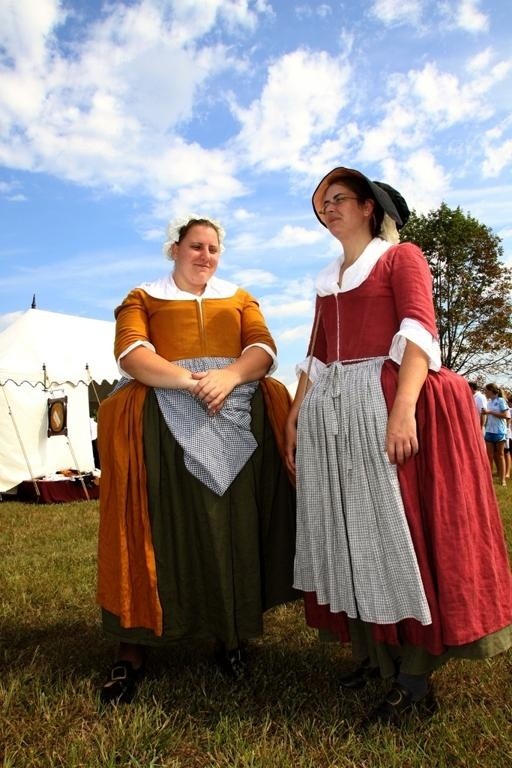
[162,213,225,262]
[311,166,410,227]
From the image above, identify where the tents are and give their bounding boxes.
[0,294,122,494]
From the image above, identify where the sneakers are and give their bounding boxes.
[335,667,380,689]
[100,660,137,706]
[213,649,265,696]
[344,682,441,739]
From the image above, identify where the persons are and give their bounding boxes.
[467,381,512,487]
[282,166,512,729]
[96,211,293,706]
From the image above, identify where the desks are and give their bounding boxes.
[23,472,101,505]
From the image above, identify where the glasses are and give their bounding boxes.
[320,195,351,214]
